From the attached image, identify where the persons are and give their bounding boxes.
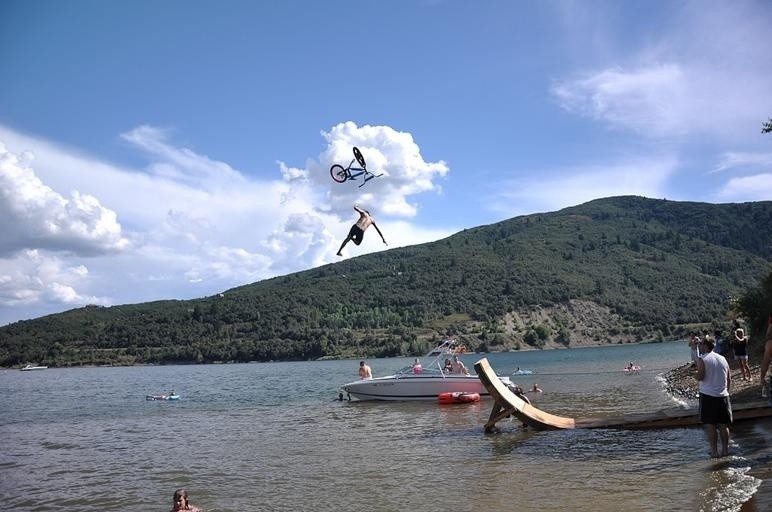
[411,358,423,374]
[765,314,772,338]
[759,339,771,387]
[451,355,464,375]
[444,358,453,371]
[358,361,373,380]
[336,205,388,257]
[333,392,348,402]
[625,361,639,371]
[528,383,542,393]
[688,329,726,370]
[150,391,176,400]
[679,336,733,458]
[512,386,532,426]
[169,488,201,511]
[462,367,469,375]
[732,327,752,381]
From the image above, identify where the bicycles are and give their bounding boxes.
[330,146,384,188]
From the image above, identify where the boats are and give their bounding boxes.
[341,364,511,401]
[20,364,48,371]
[145,395,181,400]
